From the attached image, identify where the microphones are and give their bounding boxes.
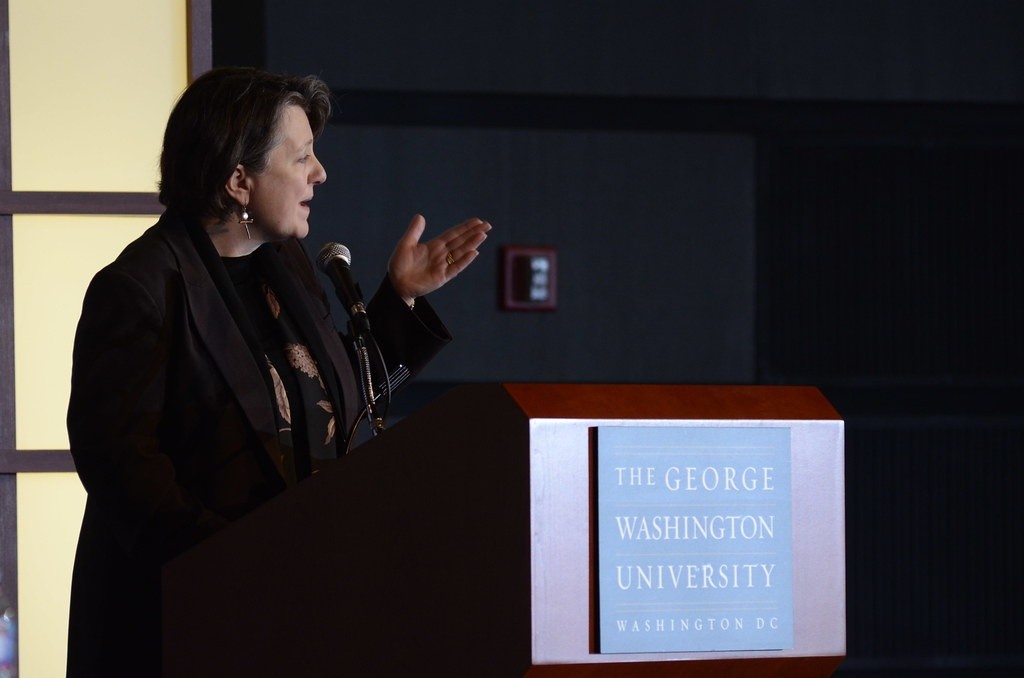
[345,366,411,456]
[316,243,371,334]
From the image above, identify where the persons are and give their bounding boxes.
[65,68,493,678]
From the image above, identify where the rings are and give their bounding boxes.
[447,253,455,265]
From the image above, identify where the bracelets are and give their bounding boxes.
[410,305,414,311]
